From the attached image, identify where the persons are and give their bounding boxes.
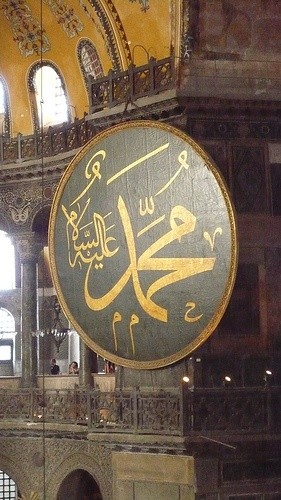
[50,359,60,374]
[68,360,79,374]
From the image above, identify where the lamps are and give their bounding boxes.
[49,298,70,353]
